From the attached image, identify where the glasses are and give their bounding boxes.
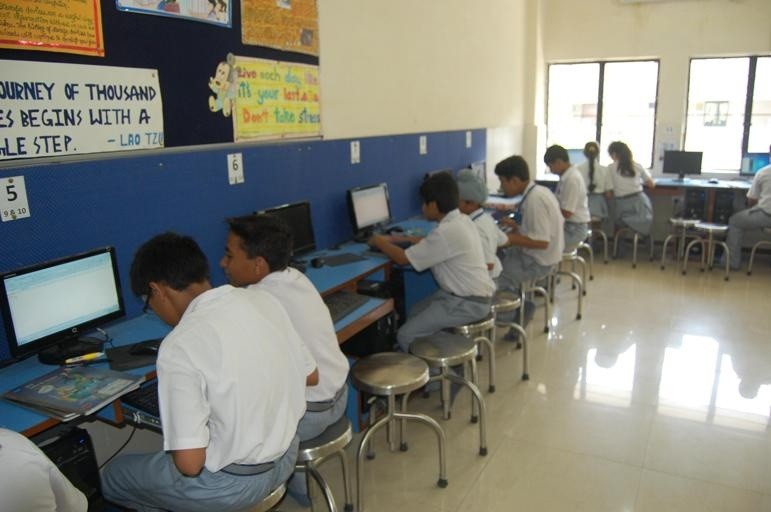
[143,288,155,315]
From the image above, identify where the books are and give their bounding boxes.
[1,362,146,425]
[362,240,412,258]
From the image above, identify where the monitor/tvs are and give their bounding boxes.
[254,200,317,265]
[349,182,392,243]
[0,248,126,365]
[470,160,489,183]
[565,147,590,165]
[663,150,703,182]
[741,151,770,174]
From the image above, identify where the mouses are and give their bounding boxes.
[311,255,324,267]
[391,223,405,234]
[131,340,158,356]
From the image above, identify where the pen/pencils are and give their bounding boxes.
[65,352,105,364]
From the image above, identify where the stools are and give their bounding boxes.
[587,214,770,280]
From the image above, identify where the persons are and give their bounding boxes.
[720,144,771,269]
[578,142,613,238]
[98,233,321,511]
[216,216,349,506]
[0,425,89,512]
[453,170,507,297]
[606,142,655,247]
[488,155,563,342]
[368,172,495,407]
[545,144,589,253]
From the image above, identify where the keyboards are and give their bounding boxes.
[320,293,372,324]
[126,382,161,415]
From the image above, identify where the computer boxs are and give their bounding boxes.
[713,193,730,252]
[39,426,104,512]
[355,281,404,354]
[682,189,705,252]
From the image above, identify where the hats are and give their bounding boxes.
[456,169,485,204]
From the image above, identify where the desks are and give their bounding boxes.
[534,168,752,260]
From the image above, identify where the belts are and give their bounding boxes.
[758,208,770,217]
[307,385,345,412]
[451,293,492,303]
[221,450,288,476]
[616,192,640,199]
